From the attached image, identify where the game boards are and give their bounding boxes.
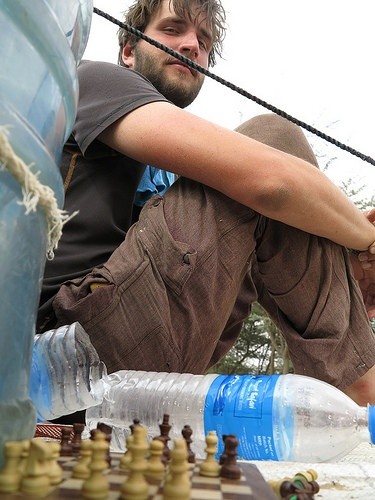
[2,439,276,500]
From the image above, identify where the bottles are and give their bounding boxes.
[29,324,107,424]
[85,370,375,465]
[1,0,90,473]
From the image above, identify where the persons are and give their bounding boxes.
[34,0,375,427]
